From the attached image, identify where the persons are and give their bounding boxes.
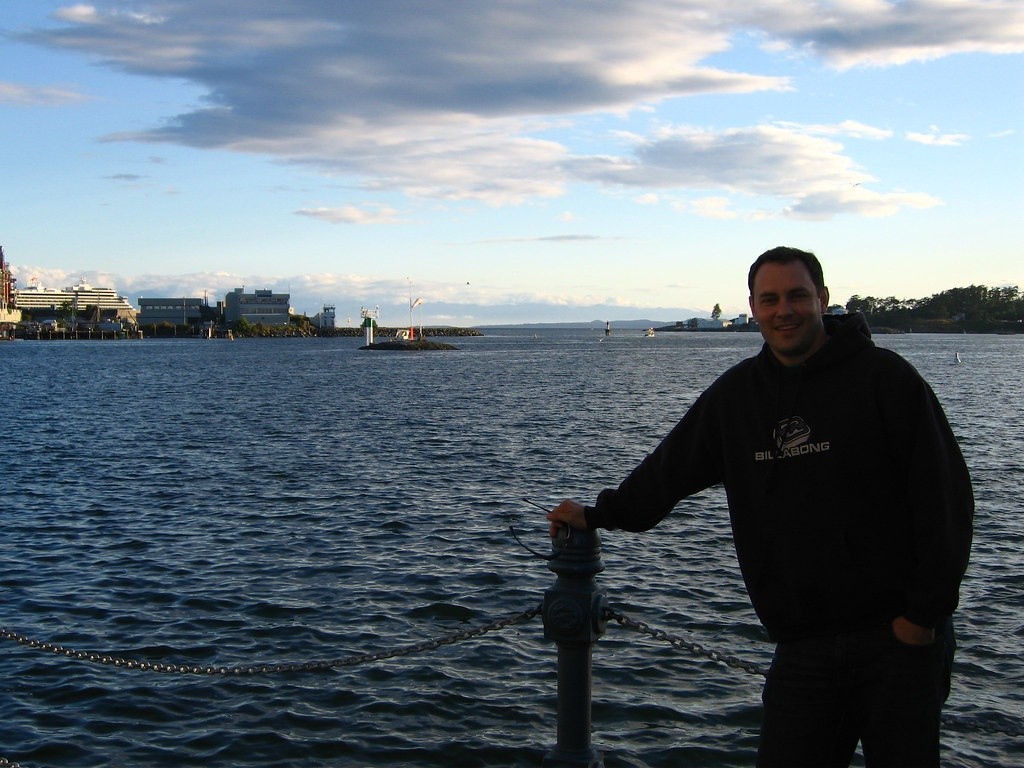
[546,247,976,768]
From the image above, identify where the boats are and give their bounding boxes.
[644,328,654,337]
